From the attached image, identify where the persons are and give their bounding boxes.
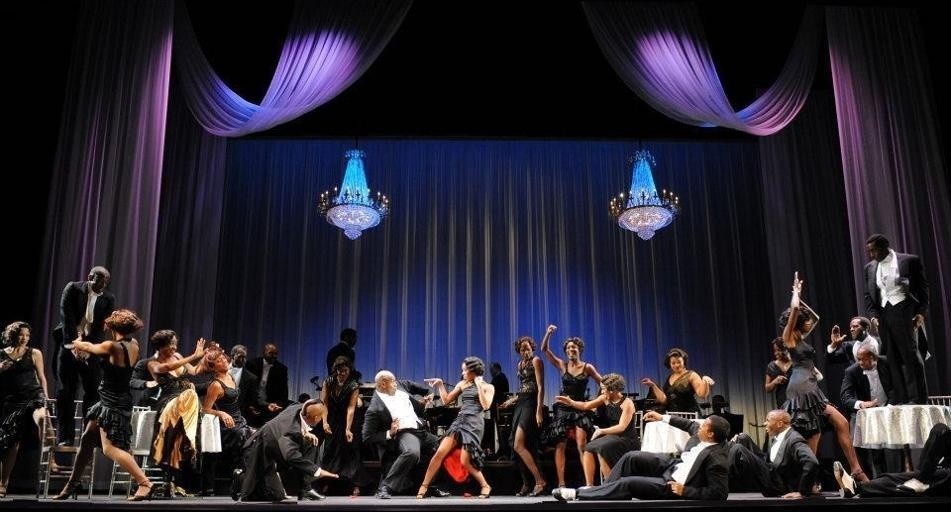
[489,272,951,499]
[416,353,495,501]
[360,369,444,500]
[0,319,49,501]
[866,233,930,405]
[53,307,154,503]
[127,328,380,504]
[54,265,117,446]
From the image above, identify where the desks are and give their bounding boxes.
[644,418,706,453]
[855,405,949,473]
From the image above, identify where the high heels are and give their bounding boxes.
[479,483,493,499]
[529,482,550,497]
[128,480,155,502]
[416,483,428,499]
[53,480,83,500]
[0,481,10,498]
[515,482,532,496]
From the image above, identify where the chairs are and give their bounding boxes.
[109,404,150,500]
[600,410,698,486]
[43,400,94,499]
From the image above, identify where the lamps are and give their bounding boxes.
[308,120,393,245]
[604,124,689,249]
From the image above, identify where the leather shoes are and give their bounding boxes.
[551,485,577,500]
[429,486,450,496]
[376,486,392,499]
[833,461,859,497]
[896,480,934,497]
[229,468,244,501]
[303,489,326,500]
